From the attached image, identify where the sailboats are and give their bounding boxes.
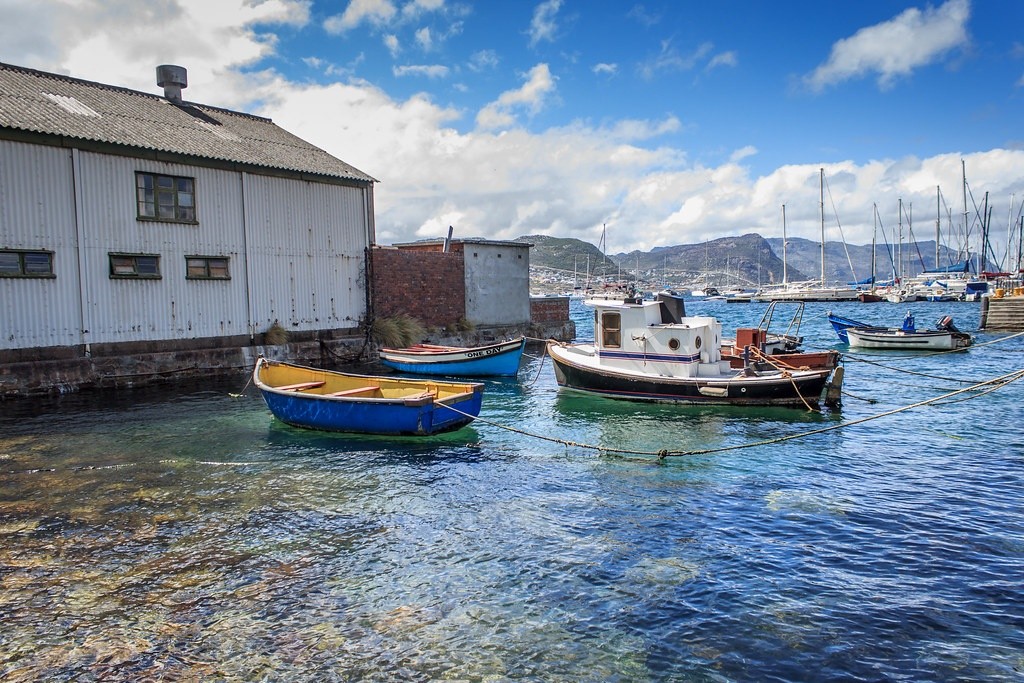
[557,159,1023,303]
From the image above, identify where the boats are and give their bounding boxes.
[826,309,977,352]
[378,333,527,378]
[252,351,486,436]
[546,291,842,407]
